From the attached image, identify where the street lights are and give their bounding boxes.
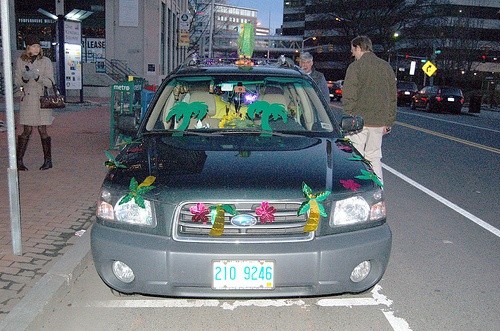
[386,31,400,61]
[302,37,316,53]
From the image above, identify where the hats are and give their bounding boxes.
[25,35,40,45]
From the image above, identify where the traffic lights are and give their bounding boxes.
[481,55,486,59]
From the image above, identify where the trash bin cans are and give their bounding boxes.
[468,94,481,112]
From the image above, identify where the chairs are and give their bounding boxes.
[178,92,221,129]
[254,94,288,125]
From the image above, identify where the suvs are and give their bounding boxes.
[90,52,393,301]
[397,81,419,106]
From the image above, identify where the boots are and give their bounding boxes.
[40,136,52,171]
[16,134,28,171]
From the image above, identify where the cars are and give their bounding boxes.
[409,85,465,114]
[326,80,342,102]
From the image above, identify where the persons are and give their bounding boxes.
[16,39,56,171]
[341,36,397,191]
[300,52,330,106]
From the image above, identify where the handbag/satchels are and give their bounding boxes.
[40,80,66,109]
